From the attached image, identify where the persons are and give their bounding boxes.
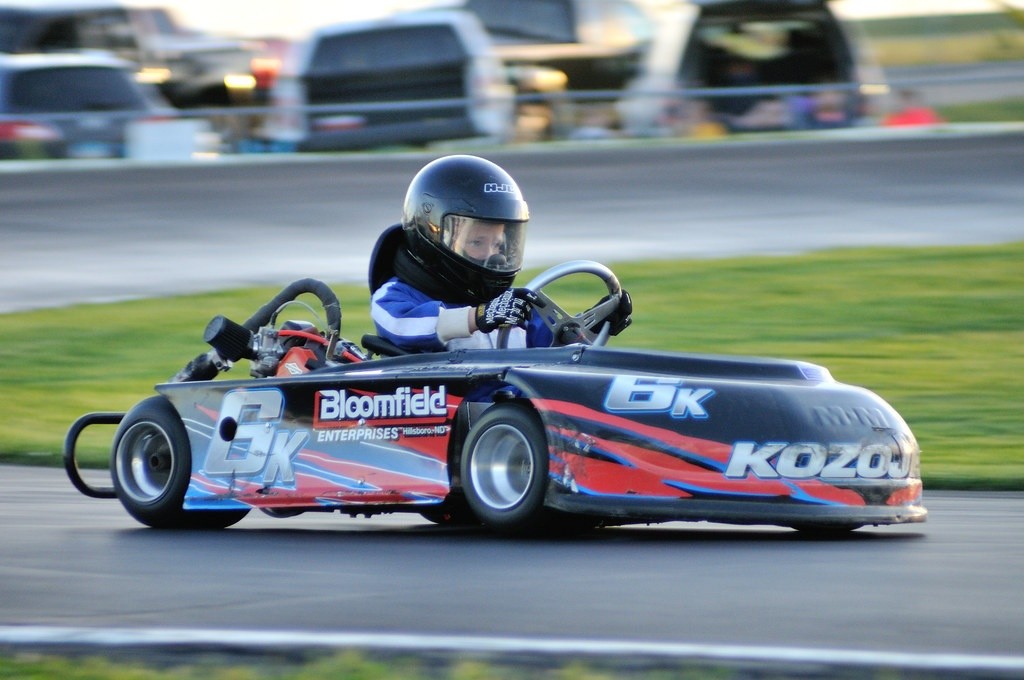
[368,155,633,361]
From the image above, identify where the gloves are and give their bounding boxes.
[575,290,632,337]
[474,288,545,334]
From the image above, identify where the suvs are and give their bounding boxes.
[613,0,893,138]
[277,10,516,152]
[0,51,184,164]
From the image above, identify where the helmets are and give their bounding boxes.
[401,154,530,301]
